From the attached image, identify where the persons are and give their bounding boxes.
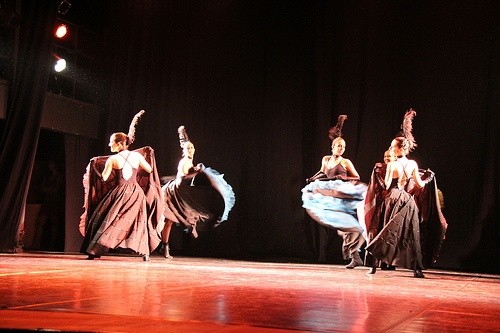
[302,109,447,278]
[78,110,165,261]
[159,125,236,261]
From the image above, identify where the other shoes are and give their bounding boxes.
[185,228,192,239]
[346,251,363,268]
[143,254,151,261]
[414,269,424,278]
[367,266,376,274]
[164,242,170,257]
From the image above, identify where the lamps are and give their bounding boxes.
[50,51,67,73]
[54,0,71,18]
[52,20,70,41]
